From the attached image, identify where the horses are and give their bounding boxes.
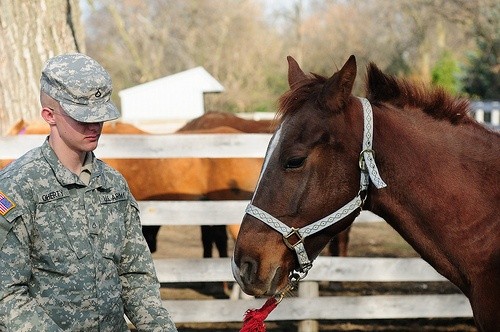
[0,111,350,292]
[231,56,500,332]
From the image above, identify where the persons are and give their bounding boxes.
[141,225,231,259]
[0,52,178,332]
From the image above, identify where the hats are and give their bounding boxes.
[39,53,121,123]
